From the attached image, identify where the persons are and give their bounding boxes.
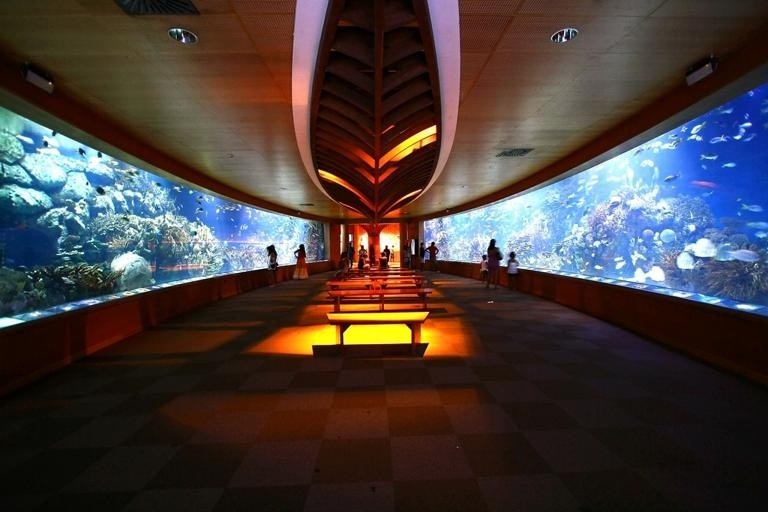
[266,244,280,288]
[506,252,520,291]
[420,242,429,272]
[346,240,371,270]
[480,255,489,284]
[292,244,309,280]
[380,242,395,269]
[425,242,439,272]
[485,238,503,290]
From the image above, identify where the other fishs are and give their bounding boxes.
[14,129,325,284]
[422,84,767,287]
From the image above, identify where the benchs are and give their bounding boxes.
[326,256,433,313]
[326,308,431,352]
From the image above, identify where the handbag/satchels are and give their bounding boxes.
[271,263,278,268]
